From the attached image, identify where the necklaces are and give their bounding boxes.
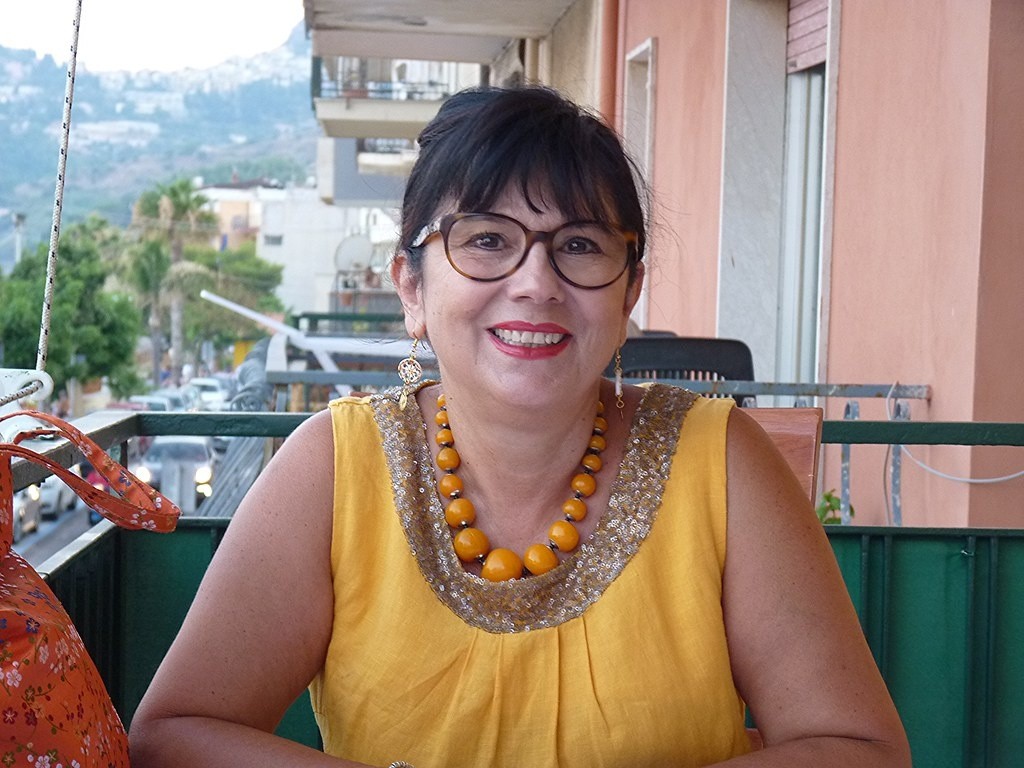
[435,395,605,581]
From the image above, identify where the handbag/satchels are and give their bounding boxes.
[0,411,183,768]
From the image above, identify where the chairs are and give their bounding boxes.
[605,337,758,409]
[740,403,825,507]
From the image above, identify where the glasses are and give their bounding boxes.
[409,210,640,293]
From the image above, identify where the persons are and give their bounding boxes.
[127,86,912,768]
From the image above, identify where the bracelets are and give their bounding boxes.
[389,761,412,768]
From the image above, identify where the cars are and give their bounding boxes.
[12,371,237,543]
